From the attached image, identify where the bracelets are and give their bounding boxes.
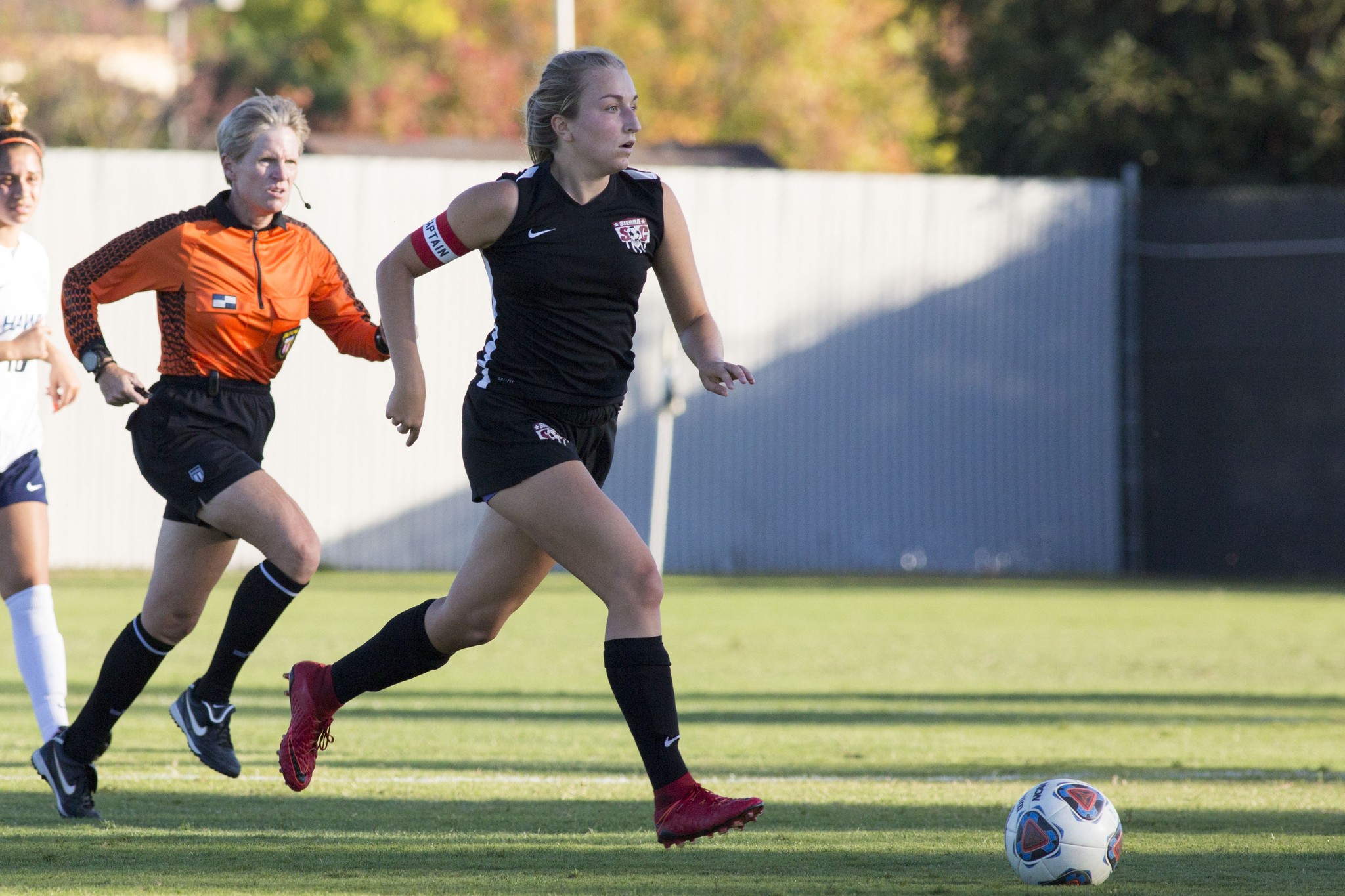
[376,332,390,354]
[94,360,117,383]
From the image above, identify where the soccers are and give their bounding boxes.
[1004,777,1121,888]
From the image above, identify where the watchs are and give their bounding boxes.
[81,344,112,373]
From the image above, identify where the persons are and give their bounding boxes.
[273,47,768,850]
[0,88,81,745]
[28,87,392,825]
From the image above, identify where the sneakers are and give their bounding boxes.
[168,680,241,781]
[650,774,763,850]
[28,727,101,828]
[278,660,341,794]
